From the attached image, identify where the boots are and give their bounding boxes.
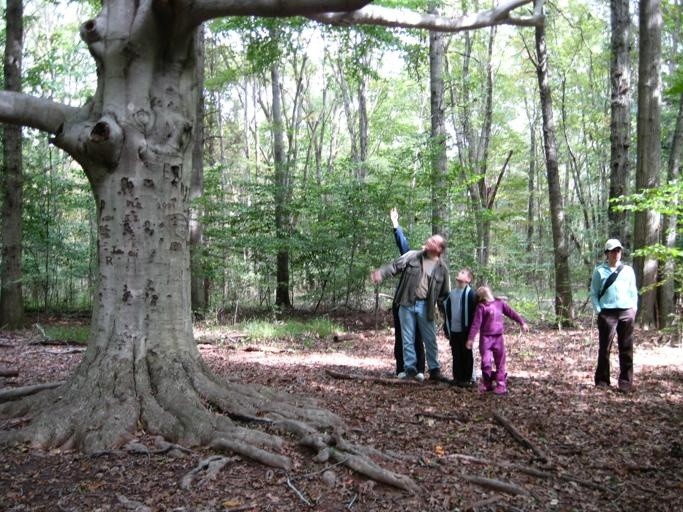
[427,368,448,383]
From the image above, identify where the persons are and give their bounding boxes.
[442,265,479,388]
[591,238,638,393]
[388,206,425,381]
[466,286,529,395]
[370,234,451,383]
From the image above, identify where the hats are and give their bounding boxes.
[604,238,624,252]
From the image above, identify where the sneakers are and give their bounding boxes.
[449,378,477,389]
[396,366,425,383]
[618,381,631,392]
[596,382,608,391]
[478,381,506,395]
